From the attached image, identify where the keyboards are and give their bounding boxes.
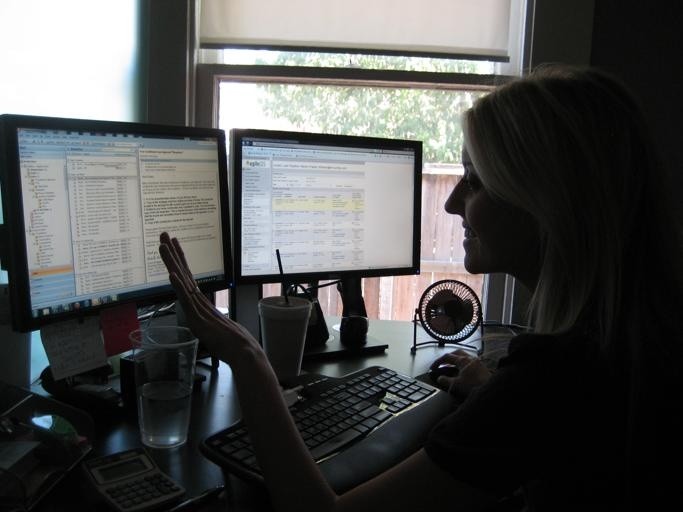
[199,366,452,496]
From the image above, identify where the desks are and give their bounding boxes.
[0,317,520,510]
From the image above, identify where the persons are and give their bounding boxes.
[158,61,682,511]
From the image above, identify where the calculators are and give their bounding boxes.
[81,446,187,511]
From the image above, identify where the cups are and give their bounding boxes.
[128,326,199,449]
[259,295,314,383]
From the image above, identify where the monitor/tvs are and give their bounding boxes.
[0,113,233,405]
[230,129,423,360]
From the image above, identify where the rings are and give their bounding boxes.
[189,285,201,296]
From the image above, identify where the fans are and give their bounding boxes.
[410,279,485,356]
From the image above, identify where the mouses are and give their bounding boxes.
[415,364,459,380]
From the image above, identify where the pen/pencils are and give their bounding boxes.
[164,485,225,512]
